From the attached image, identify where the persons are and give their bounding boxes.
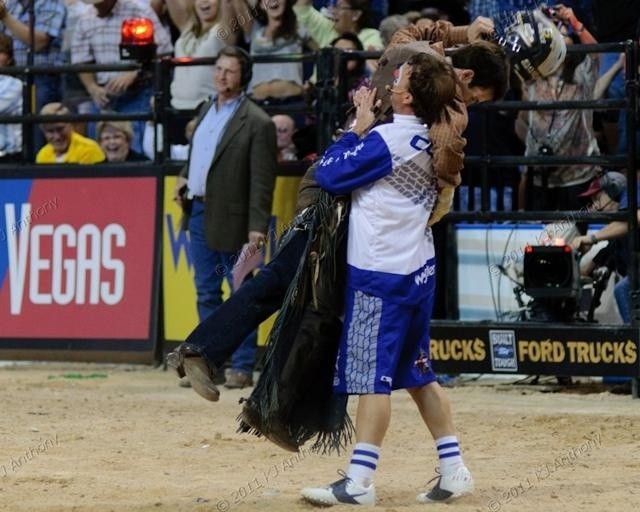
[569,170,640,327]
[301,49,475,508]
[173,45,278,390]
[166,12,512,456]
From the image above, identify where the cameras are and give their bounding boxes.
[543,6,560,18]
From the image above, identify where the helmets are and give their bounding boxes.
[491,6,569,85]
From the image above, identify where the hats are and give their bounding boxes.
[580,169,628,204]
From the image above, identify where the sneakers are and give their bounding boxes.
[298,472,378,507]
[415,463,477,506]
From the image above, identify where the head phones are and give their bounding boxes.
[605,172,625,203]
[236,45,253,85]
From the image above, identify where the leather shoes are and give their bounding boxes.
[238,404,301,455]
[163,342,254,403]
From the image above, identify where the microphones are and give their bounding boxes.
[385,84,410,94]
[596,199,613,211]
[226,85,246,93]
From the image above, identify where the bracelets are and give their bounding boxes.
[591,235,600,244]
